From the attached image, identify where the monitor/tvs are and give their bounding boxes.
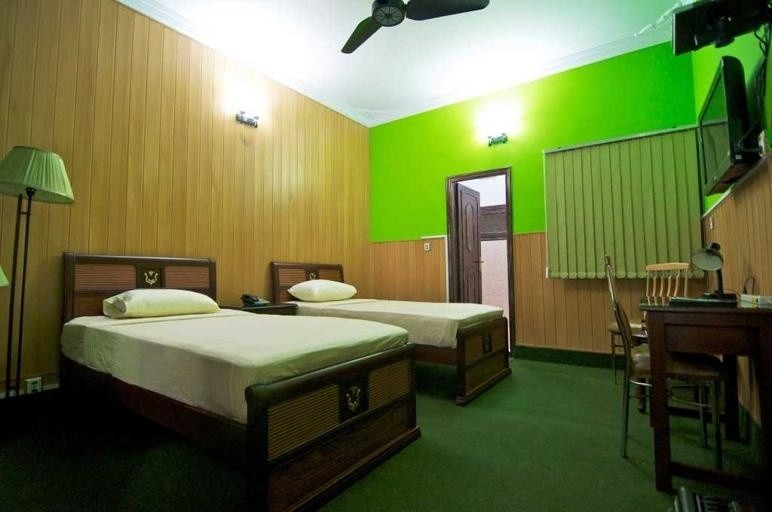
[699,56,758,196]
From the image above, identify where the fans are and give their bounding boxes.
[341,0,490,54]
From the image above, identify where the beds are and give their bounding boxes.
[272,262,511,406]
[60,252,420,512]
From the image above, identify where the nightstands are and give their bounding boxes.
[219,300,297,316]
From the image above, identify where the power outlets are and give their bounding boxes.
[26,377,42,395]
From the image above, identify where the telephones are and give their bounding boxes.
[240,294,270,307]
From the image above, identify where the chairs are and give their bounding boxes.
[606,262,690,386]
[604,256,726,468]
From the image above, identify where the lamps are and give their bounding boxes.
[691,242,737,299]
[0,146,75,398]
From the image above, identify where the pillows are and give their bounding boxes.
[287,279,358,302]
[103,288,221,318]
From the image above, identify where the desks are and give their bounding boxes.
[639,302,772,492]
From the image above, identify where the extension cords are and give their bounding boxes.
[739,294,772,304]
